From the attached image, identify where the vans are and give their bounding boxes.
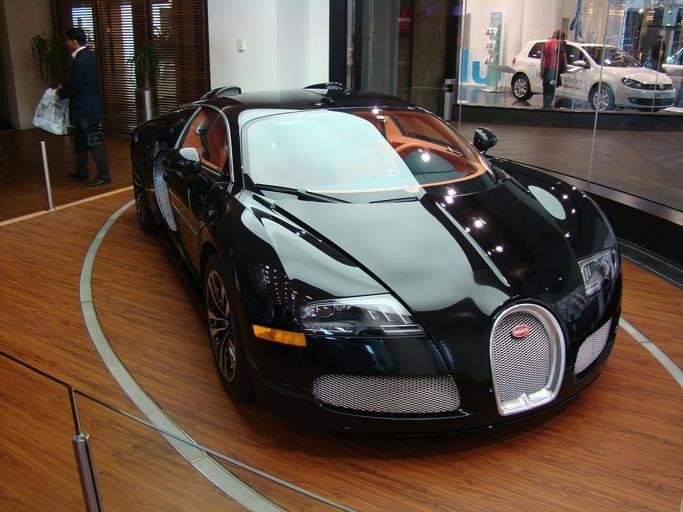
[510,38,677,113]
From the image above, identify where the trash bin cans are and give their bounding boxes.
[443,79,455,121]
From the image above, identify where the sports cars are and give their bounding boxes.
[126,82,621,440]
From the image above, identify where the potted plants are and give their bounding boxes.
[127,25,172,125]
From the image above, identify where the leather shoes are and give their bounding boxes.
[86,176,111,186]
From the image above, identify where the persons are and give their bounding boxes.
[55,28,111,189]
[652,36,667,70]
[540,28,569,108]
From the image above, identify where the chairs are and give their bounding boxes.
[333,112,398,182]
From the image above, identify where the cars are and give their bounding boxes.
[663,48,683,108]
